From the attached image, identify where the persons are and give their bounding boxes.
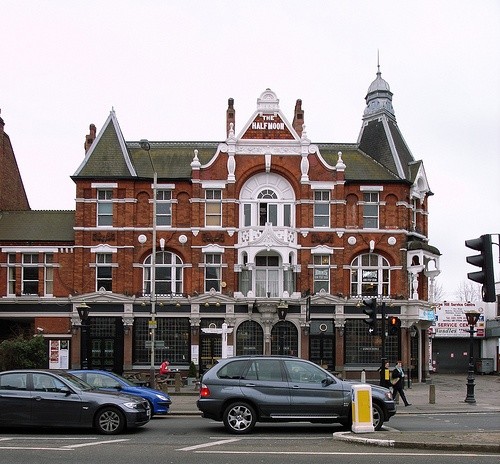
[377,361,393,388]
[392,360,412,407]
[160,360,170,380]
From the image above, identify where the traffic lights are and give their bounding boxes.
[362,298,377,335]
[465,233,496,303]
[388,316,398,336]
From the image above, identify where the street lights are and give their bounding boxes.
[464,309,481,403]
[140,138,159,366]
[275,305,289,354]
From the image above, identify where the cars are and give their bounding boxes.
[66,369,172,419]
[0,369,153,434]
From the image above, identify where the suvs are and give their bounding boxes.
[196,355,397,434]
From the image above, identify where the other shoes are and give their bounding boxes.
[405,403,412,407]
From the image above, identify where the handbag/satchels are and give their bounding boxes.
[390,368,401,385]
